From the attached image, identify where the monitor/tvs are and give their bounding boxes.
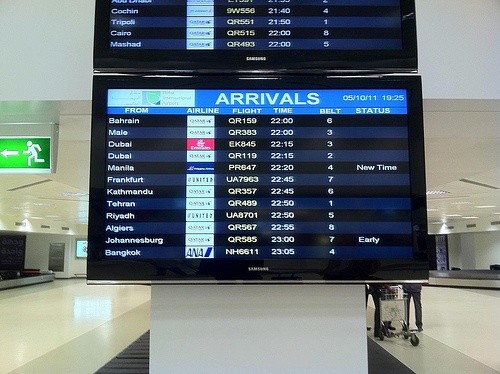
[86,74,430,284]
[76,238,88,258]
[93,0,418,74]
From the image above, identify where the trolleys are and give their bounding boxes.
[373,284,420,347]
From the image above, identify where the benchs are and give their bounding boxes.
[74,273,87,278]
[20,269,41,276]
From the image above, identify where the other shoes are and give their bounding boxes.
[389,326,396,330]
[367,326,371,330]
[418,326,423,331]
[374,333,380,336]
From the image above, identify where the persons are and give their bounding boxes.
[367,285,398,338]
[402,284,423,331]
